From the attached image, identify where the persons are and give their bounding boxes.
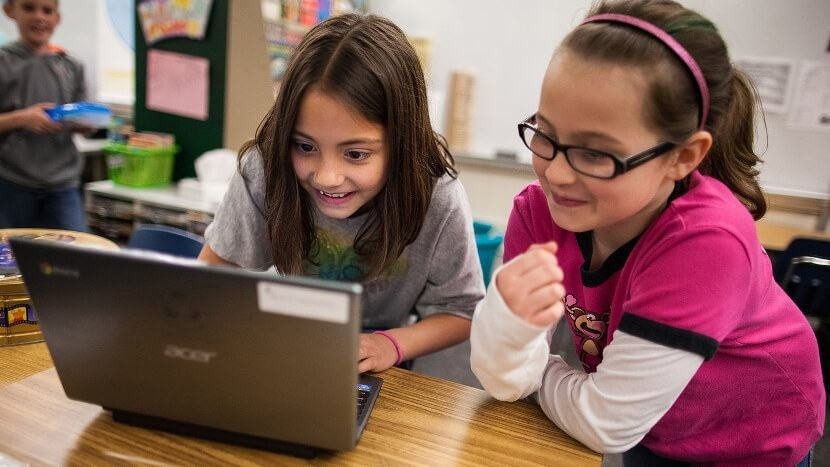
[198,14,486,374]
[470,0,825,467]
[0,0,110,233]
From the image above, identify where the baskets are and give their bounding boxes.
[103,139,177,187]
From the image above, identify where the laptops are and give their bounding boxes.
[8,236,383,452]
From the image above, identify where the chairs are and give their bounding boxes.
[776,231,830,348]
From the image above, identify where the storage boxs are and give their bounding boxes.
[105,143,179,186]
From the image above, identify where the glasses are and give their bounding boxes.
[517,112,680,179]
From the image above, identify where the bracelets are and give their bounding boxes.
[372,330,402,366]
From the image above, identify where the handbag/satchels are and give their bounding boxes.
[777,237,830,317]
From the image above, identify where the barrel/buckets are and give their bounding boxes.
[474,222,502,285]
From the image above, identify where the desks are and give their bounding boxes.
[0,132,601,466]
[753,211,830,250]
